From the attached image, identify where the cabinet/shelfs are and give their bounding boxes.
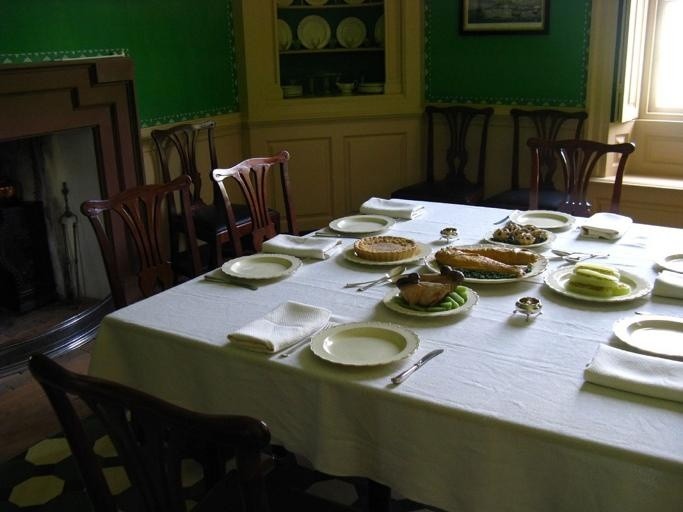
[235,0,423,231]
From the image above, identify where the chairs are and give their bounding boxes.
[484,108,588,210]
[29,354,273,512]
[391,106,494,204]
[151,119,280,277]
[526,135,635,221]
[80,173,203,309]
[210,148,300,258]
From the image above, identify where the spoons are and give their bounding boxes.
[551,249,590,257]
[351,265,407,291]
[563,255,609,263]
[346,274,403,288]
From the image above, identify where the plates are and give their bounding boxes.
[328,214,397,234]
[653,247,683,273]
[542,263,655,303]
[221,252,303,279]
[484,227,553,247]
[510,211,574,229]
[342,242,432,266]
[424,243,549,284]
[382,285,479,317]
[612,314,683,358]
[310,320,420,367]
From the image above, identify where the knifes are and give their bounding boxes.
[315,232,363,239]
[204,275,257,292]
[391,349,444,384]
[492,215,510,225]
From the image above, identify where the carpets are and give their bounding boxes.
[0,409,438,512]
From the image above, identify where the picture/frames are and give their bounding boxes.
[459,0,550,36]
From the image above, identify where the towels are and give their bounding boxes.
[583,341,683,402]
[652,269,683,298]
[227,300,333,356]
[359,197,425,221]
[262,233,343,260]
[581,212,633,240]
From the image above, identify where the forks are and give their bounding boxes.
[280,322,337,357]
